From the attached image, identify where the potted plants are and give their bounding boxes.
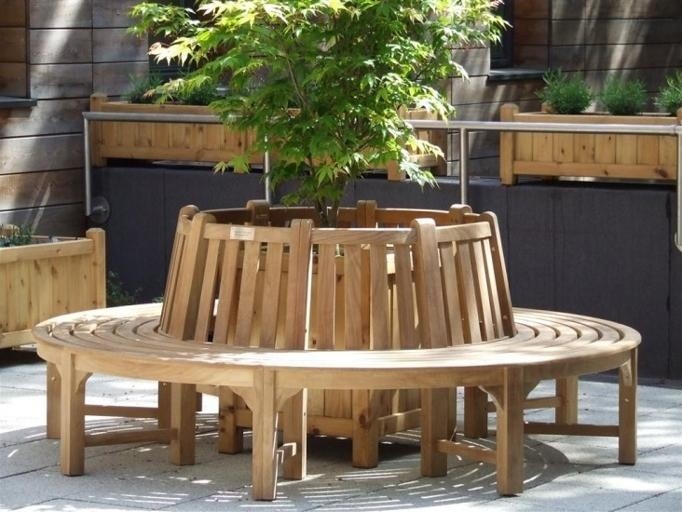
[123,0,511,470]
[0,218,109,351]
[85,73,267,172]
[491,66,677,187]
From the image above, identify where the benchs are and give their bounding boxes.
[27,292,644,502]
